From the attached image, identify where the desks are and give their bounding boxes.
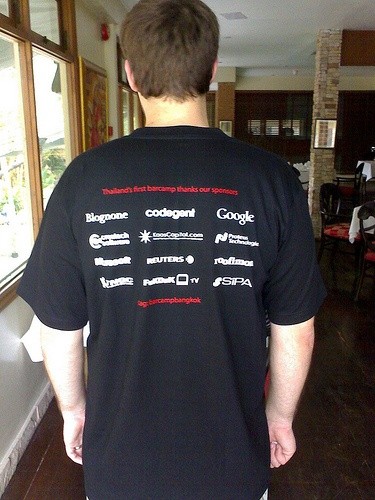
[356,161,375,195]
[333,162,364,208]
[348,206,375,282]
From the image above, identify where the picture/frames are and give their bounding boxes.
[313,118,338,149]
[79,55,110,152]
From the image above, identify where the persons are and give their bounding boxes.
[17,0,328,500]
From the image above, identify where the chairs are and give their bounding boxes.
[317,183,364,287]
[351,199,375,303]
